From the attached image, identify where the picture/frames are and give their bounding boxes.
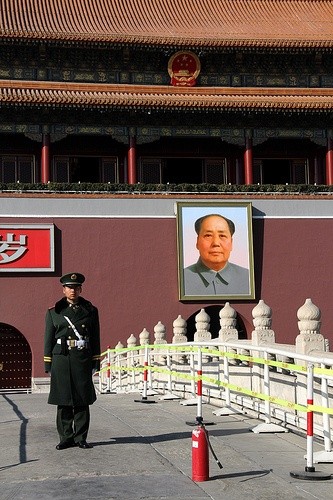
[176,202,255,301]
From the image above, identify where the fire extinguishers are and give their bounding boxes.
[192,416,223,482]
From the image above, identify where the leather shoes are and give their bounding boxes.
[55,441,93,450]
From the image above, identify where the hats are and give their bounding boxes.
[60,272,85,287]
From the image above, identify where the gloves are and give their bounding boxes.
[48,370,51,376]
[92,369,97,374]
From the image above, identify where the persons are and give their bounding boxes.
[43,272,100,450]
[183,214,250,295]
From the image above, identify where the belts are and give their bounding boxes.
[57,339,85,347]
